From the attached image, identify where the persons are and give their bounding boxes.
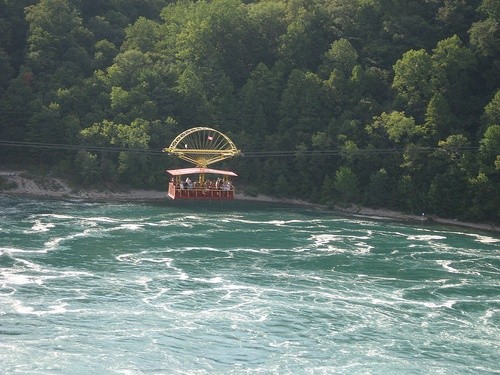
[171,178,232,191]
